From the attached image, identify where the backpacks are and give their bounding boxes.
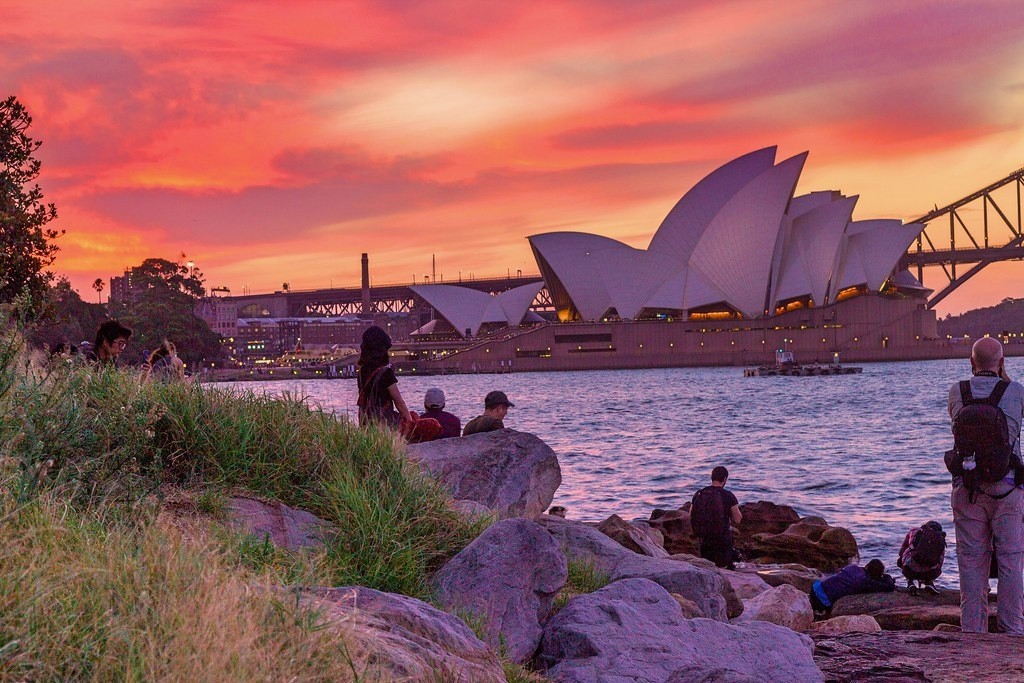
[363,391,390,428]
[906,527,947,566]
[944,380,1023,504]
[690,486,730,538]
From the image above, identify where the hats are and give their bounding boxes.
[485,391,517,409]
[921,520,942,531]
[359,326,393,351]
[425,387,445,408]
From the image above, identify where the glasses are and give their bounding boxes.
[112,340,128,349]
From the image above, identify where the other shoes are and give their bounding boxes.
[924,581,940,594]
[907,581,918,589]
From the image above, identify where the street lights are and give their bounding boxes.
[187,260,196,350]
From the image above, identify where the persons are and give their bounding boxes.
[944,337,1024,635]
[82,320,151,375]
[549,506,567,519]
[419,388,461,438]
[462,390,516,436]
[810,558,895,614]
[897,520,948,596]
[689,466,743,570]
[150,342,184,387]
[356,326,443,445]
